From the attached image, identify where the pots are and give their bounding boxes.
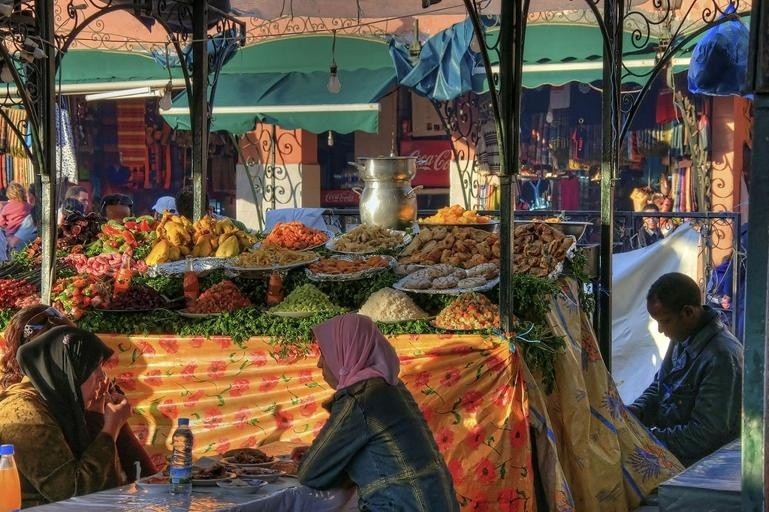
[348,150,423,230]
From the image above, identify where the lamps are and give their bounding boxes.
[159,43,175,112]
[326,29,342,95]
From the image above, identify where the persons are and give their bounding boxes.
[296,314,461,512]
[57,185,248,238]
[638,204,664,248]
[625,272,743,468]
[705,222,748,345]
[0,181,38,263]
[0,304,158,509]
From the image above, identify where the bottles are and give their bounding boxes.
[0,444,21,511]
[170,418,193,496]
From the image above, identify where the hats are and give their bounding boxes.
[152,196,178,214]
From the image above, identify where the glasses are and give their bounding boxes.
[101,196,135,210]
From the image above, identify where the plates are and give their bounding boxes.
[136,455,285,494]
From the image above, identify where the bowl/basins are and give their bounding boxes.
[413,220,499,232]
[512,220,594,243]
